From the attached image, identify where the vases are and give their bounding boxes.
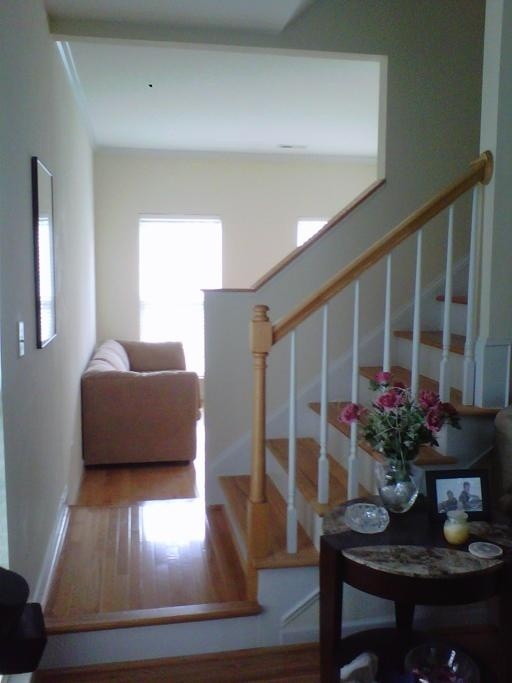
[378,467,419,514]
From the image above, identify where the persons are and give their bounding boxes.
[458,481,479,510]
[438,489,457,513]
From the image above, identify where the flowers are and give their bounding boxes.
[337,369,458,471]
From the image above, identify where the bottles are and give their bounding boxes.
[443,510,469,546]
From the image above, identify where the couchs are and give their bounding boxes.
[80,340,199,466]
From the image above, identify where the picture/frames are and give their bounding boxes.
[32,157,57,351]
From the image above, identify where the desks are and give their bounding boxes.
[319,490,512,679]
